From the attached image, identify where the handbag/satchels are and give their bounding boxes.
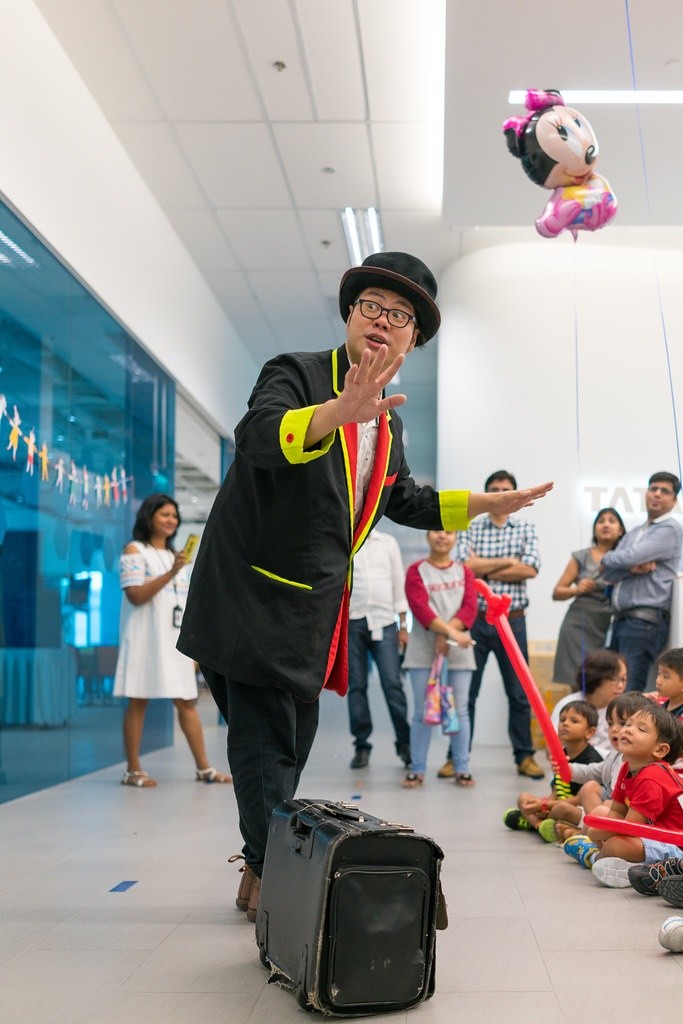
[423,652,459,735]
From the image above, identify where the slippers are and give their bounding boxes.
[402,774,422,790]
[455,773,473,787]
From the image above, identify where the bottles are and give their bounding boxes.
[441,686,459,735]
[423,679,441,724]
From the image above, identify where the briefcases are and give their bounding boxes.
[254,798,451,1017]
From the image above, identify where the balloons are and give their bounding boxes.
[503,87,618,243]
[583,814,683,848]
[476,577,572,785]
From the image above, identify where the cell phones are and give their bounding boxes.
[181,534,200,563]
[446,639,476,647]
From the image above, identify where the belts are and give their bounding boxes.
[479,609,526,617]
[618,606,666,626]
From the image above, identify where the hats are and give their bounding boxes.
[338,252,441,347]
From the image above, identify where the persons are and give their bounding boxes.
[505,648,683,954]
[600,471,683,693]
[551,507,626,695]
[110,493,233,788]
[398,530,476,789]
[176,249,555,915]
[345,528,412,769]
[436,471,544,780]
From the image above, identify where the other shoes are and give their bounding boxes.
[519,757,546,779]
[231,854,262,920]
[438,760,456,778]
[349,747,369,767]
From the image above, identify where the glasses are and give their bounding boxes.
[352,299,418,329]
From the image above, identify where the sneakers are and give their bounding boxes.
[658,874,683,907]
[659,916,683,953]
[627,858,683,896]
[563,836,599,869]
[505,809,535,831]
[538,818,558,842]
[591,857,643,888]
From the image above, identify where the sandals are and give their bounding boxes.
[198,767,232,784]
[124,770,156,787]
[554,821,581,842]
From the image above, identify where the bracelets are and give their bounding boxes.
[400,622,408,630]
[542,797,547,811]
